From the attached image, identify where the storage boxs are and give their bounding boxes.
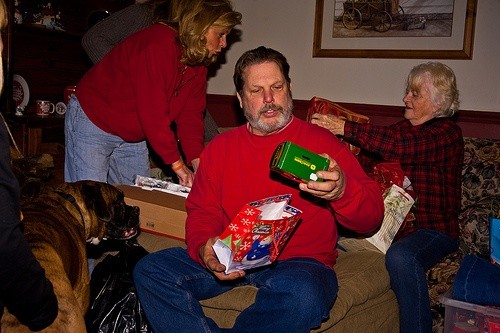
[436,293,500,333]
[116,183,189,242]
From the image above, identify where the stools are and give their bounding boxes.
[200,248,401,333]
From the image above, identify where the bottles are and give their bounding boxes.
[15,0,23,24]
[63,81,77,105]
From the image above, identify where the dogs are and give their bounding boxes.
[0,180,141,333]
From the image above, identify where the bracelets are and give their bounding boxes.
[170,159,185,170]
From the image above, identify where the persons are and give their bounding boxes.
[334,62,465,333]
[134,46,385,333]
[65,1,241,183]
[83,0,219,146]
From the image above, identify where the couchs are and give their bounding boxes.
[425,139,500,333]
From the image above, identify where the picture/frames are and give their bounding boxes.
[312,0,477,60]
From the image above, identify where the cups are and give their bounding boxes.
[36,99,54,116]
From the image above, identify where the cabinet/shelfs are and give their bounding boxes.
[8,0,138,181]
